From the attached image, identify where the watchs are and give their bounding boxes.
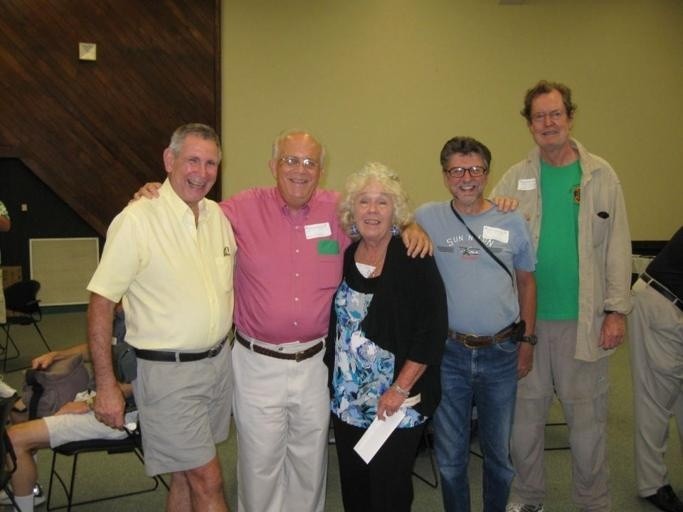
[521,334,537,345]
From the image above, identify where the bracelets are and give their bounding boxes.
[391,383,410,397]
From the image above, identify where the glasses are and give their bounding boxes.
[443,166,486,178]
[280,156,317,170]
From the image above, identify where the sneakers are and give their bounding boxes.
[0,481,46,509]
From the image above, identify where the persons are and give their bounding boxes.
[322,164,449,512]
[409,135,538,512]
[86,123,239,512]
[0,200,11,325]
[133,126,434,512]
[628,226,683,512]
[6,297,139,512]
[485,79,633,512]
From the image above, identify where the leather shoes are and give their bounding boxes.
[644,483,683,511]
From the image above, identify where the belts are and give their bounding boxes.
[237,337,326,362]
[639,270,683,311]
[446,328,517,348]
[134,342,227,364]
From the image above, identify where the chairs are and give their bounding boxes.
[45,421,160,511]
[0,278,53,373]
